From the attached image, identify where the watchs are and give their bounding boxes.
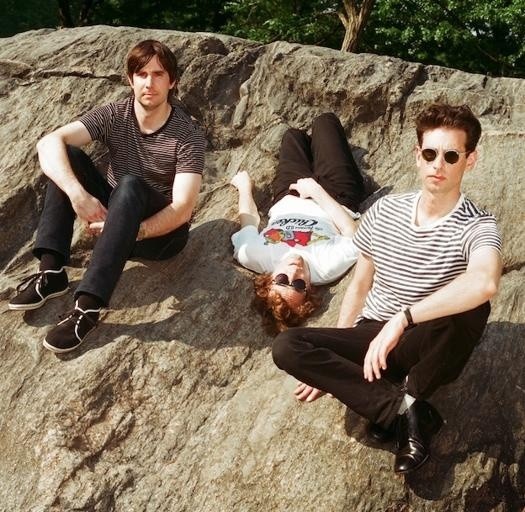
[400,305,418,331]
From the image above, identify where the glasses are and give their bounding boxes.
[269,273,308,291]
[416,145,469,165]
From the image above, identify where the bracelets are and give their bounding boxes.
[135,223,145,242]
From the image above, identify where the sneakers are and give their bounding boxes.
[41,299,103,353]
[7,265,71,312]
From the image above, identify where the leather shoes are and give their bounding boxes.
[366,393,446,477]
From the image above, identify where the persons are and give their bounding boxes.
[5,36,209,357]
[270,100,506,477]
[223,112,365,340]
